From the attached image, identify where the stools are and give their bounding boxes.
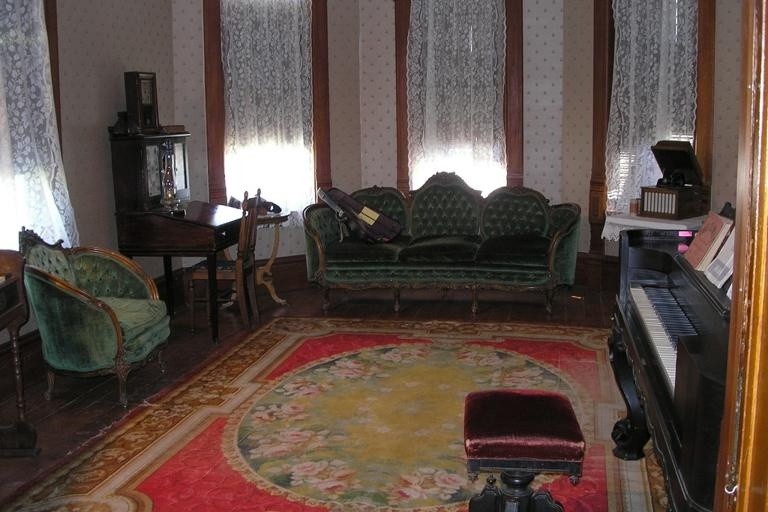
[463,389,586,511]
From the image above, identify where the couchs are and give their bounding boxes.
[301,171,581,315]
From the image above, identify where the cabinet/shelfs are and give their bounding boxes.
[108,71,249,344]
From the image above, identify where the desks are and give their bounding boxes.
[601,214,709,289]
[218,213,290,309]
[0,249,37,452]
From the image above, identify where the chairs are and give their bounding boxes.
[182,188,261,335]
[18,225,171,408]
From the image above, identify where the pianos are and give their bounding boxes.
[607,203,734,510]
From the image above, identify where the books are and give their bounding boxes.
[683,211,737,300]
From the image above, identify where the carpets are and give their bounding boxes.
[0,317,676,512]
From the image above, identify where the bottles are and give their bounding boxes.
[630,197,641,217]
